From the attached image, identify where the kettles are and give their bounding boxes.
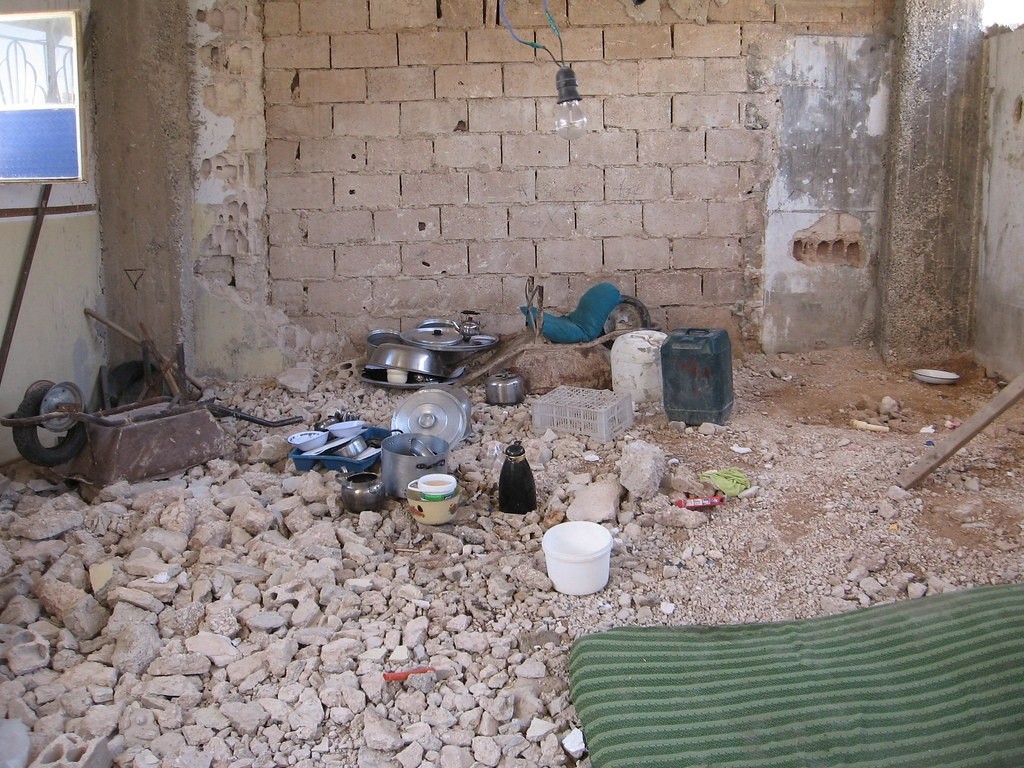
[499,440,538,513]
[485,367,526,406]
[336,467,383,514]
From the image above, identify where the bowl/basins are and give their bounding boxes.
[369,343,445,377]
[324,421,365,439]
[287,430,329,452]
[386,369,409,384]
[410,439,435,456]
[331,436,367,458]
[419,384,472,441]
[407,484,463,525]
[365,330,401,360]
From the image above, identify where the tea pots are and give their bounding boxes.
[451,309,481,335]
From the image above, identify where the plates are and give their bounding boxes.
[912,368,958,384]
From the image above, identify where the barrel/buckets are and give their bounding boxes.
[541,520,614,597]
[409,474,457,502]
[612,330,665,403]
[662,327,732,425]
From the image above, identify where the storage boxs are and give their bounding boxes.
[529,384,633,441]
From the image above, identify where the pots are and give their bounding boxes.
[380,429,450,500]
